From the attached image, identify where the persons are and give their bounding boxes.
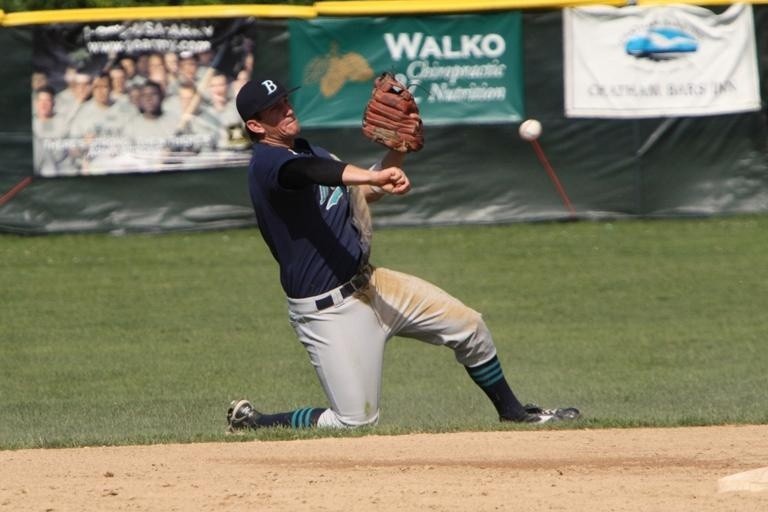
[227,78,582,429]
[32,23,255,178]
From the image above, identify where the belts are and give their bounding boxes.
[316,264,373,310]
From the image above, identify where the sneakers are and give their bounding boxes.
[226,398,262,432]
[500,403,580,424]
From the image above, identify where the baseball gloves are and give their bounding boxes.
[361,71,431,152]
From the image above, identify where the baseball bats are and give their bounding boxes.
[61,53,122,132]
[165,44,228,135]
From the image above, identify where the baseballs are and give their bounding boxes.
[519,119,542,141]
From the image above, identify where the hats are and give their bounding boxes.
[237,79,301,121]
